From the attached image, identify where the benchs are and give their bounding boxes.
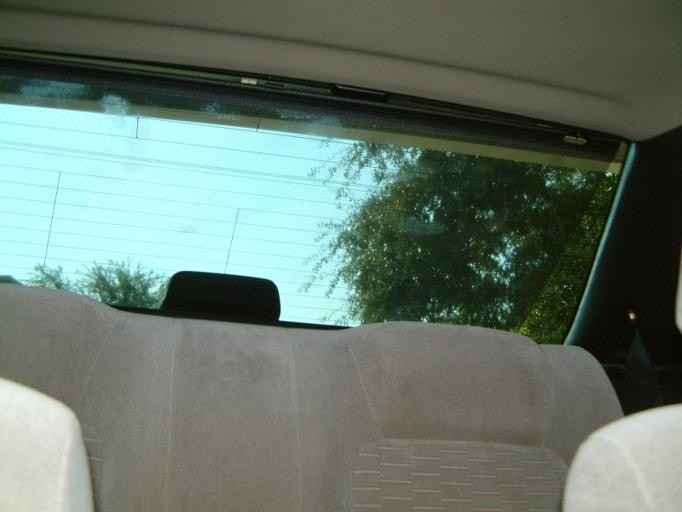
[0,284,626,510]
